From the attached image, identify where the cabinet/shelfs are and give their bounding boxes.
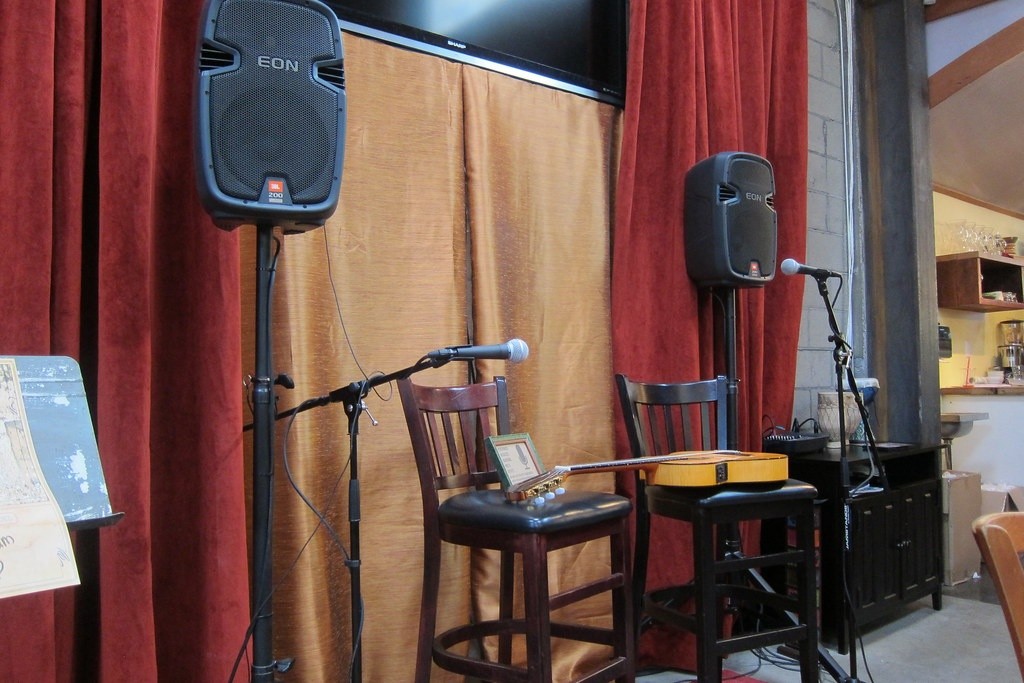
[766,440,945,655]
[935,250,1024,313]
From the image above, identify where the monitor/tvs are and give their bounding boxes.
[323,0,627,108]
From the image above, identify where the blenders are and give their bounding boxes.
[997,319,1024,385]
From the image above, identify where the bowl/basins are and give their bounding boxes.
[1003,236,1018,243]
[987,370,1003,385]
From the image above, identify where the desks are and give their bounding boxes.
[942,412,989,469]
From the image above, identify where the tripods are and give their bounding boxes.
[646,289,852,682]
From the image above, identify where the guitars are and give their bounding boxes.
[503,447,790,507]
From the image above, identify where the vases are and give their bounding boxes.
[846,392,864,446]
[816,391,851,449]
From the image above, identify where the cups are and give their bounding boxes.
[1005,242,1016,255]
[1011,365,1024,386]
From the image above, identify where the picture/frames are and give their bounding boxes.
[484,432,552,494]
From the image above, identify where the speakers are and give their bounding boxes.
[193,0,346,236]
[682,150,777,288]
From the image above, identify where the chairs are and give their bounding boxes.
[616,375,824,683]
[970,511,1024,683]
[396,375,637,683]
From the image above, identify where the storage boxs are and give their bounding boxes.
[982,484,1024,514]
[943,469,982,588]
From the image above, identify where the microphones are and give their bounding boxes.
[781,259,841,276]
[427,338,529,362]
